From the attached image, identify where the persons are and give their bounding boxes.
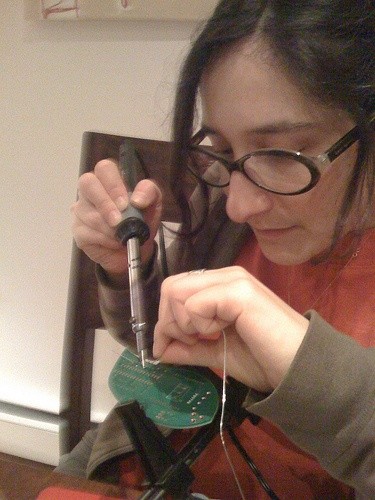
[69,1,374,499]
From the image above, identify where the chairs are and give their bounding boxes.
[62,129,218,457]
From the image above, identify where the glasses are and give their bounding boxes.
[178,113,375,196]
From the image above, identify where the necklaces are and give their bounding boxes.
[285,228,375,316]
